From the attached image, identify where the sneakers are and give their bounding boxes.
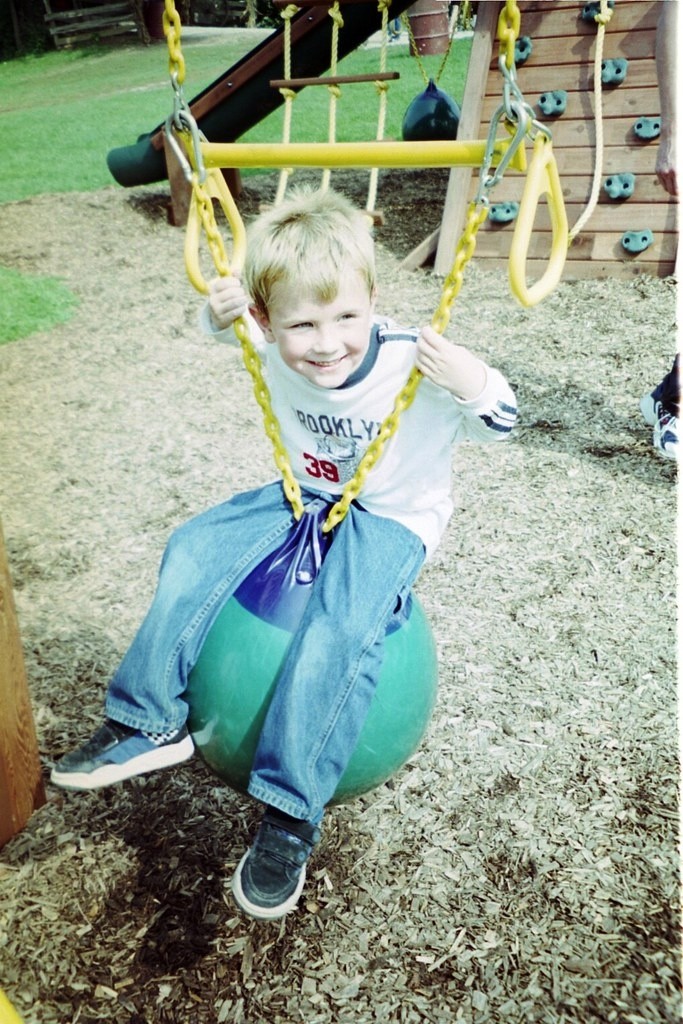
[232,805,322,921]
[641,382,680,462]
[50,718,194,790]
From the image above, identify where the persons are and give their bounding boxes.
[49,184,516,917]
[640,353,679,461]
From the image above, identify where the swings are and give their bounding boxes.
[147,0,577,820]
